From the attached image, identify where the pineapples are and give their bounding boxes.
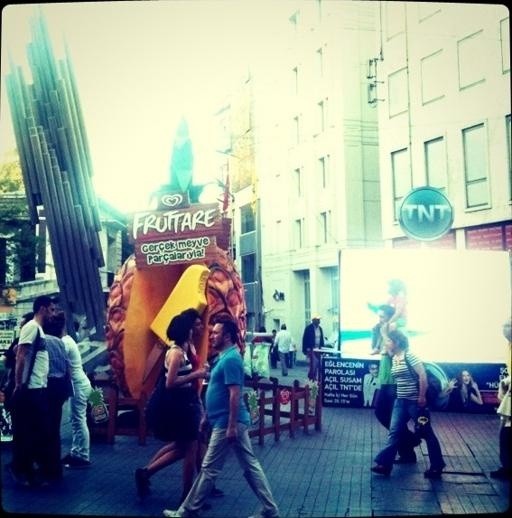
[91,403,109,424]
[106,245,246,398]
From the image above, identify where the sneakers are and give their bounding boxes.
[135,465,226,518]
[370,433,443,481]
[249,508,280,518]
[5,454,90,479]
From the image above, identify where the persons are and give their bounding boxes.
[4,296,93,483]
[145,309,228,497]
[367,281,447,480]
[301,314,324,380]
[251,325,296,376]
[134,316,209,500]
[439,369,483,413]
[489,320,512,477]
[364,363,380,409]
[164,311,279,516]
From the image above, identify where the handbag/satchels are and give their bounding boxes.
[405,348,443,401]
[143,343,197,443]
